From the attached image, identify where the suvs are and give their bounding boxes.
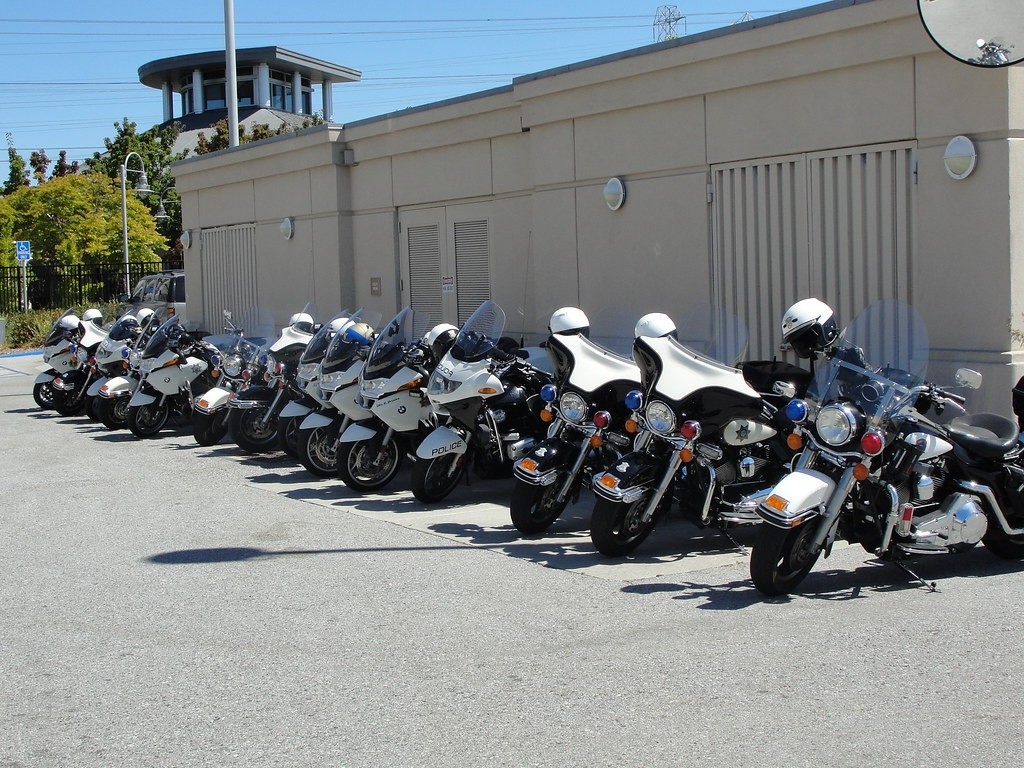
[115,268,186,326]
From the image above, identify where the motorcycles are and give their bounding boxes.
[750,294,1024,598]
[966,39,1016,65]
[509,298,644,537]
[31,295,560,506]
[590,303,818,558]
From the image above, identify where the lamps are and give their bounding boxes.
[280,217,294,239]
[180,229,192,248]
[944,137,977,179]
[602,178,627,211]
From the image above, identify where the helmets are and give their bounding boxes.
[119,314,140,327]
[428,323,459,361]
[327,318,356,338]
[635,312,677,340]
[550,306,590,339]
[137,308,159,327]
[60,314,81,331]
[782,297,839,358]
[82,309,103,327]
[344,322,374,347]
[289,313,313,333]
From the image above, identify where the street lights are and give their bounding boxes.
[121,151,156,300]
